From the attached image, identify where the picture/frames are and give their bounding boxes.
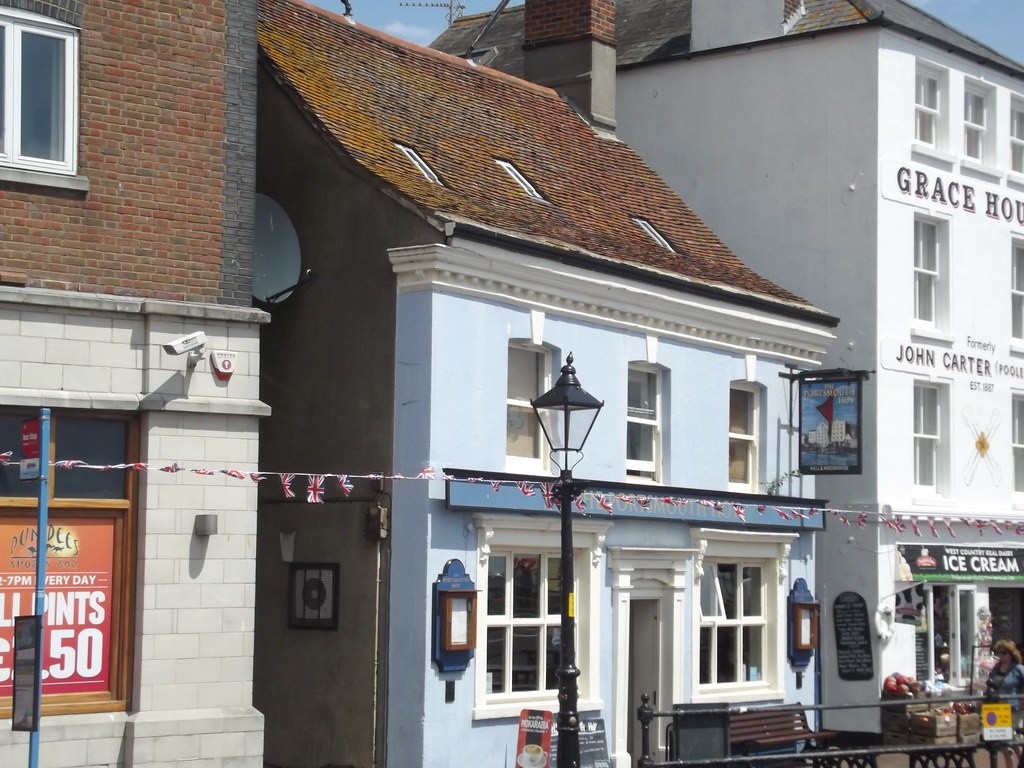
[288,562,340,632]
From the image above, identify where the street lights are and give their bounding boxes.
[528,349,604,767]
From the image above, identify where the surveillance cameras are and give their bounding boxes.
[163,331,207,356]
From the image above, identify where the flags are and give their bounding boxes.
[573,494,1024,538]
[0,450,562,515]
[894,584,925,627]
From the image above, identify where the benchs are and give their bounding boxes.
[729,702,840,755]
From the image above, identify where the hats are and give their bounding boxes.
[940,653,950,661]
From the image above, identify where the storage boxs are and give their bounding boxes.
[909,735,956,745]
[931,691,954,712]
[957,712,980,736]
[957,733,980,745]
[910,712,957,737]
[882,730,909,746]
[883,691,930,713]
[883,712,910,733]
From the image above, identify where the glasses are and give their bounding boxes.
[994,650,1006,655]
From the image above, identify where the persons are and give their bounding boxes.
[986,639,1024,768]
